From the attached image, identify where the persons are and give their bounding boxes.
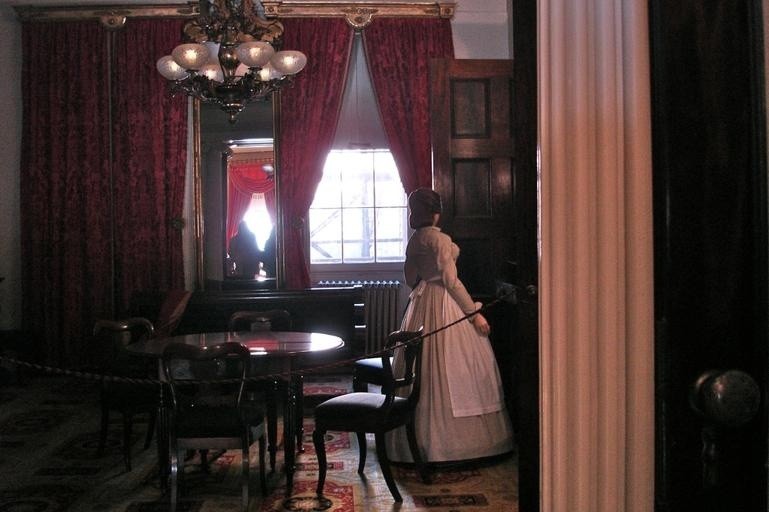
[378,186,516,469]
[259,224,277,277]
[227,221,259,281]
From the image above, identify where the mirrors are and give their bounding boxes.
[192,90,284,291]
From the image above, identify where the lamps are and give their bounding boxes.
[156,1,307,126]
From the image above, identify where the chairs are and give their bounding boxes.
[95,318,176,473]
[229,311,290,473]
[311,330,423,504]
[353,355,396,392]
[162,342,267,512]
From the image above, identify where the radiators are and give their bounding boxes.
[316,281,402,357]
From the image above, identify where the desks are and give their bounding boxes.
[124,332,345,490]
